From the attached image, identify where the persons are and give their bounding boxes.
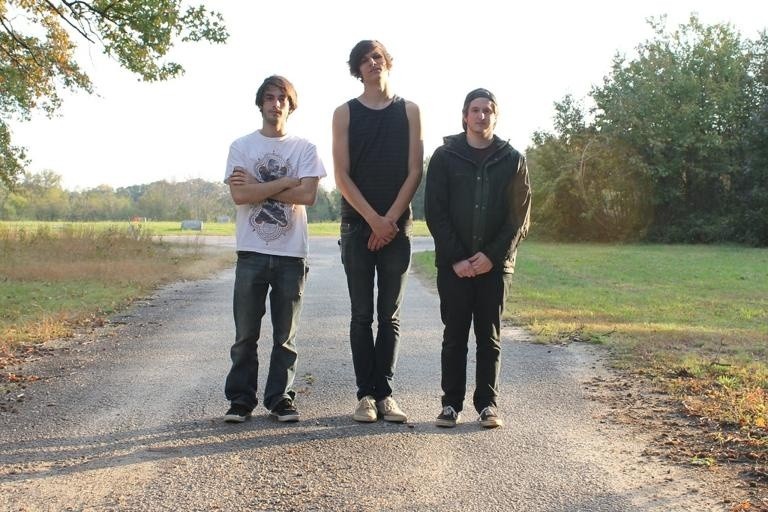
[222,73,328,424]
[422,86,535,428]
[331,39,426,426]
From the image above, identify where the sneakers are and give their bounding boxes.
[224,406,252,422]
[477,405,502,427]
[270,398,300,422]
[377,396,407,422]
[436,406,460,427]
[352,395,379,422]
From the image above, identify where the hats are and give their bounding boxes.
[462,88,499,133]
